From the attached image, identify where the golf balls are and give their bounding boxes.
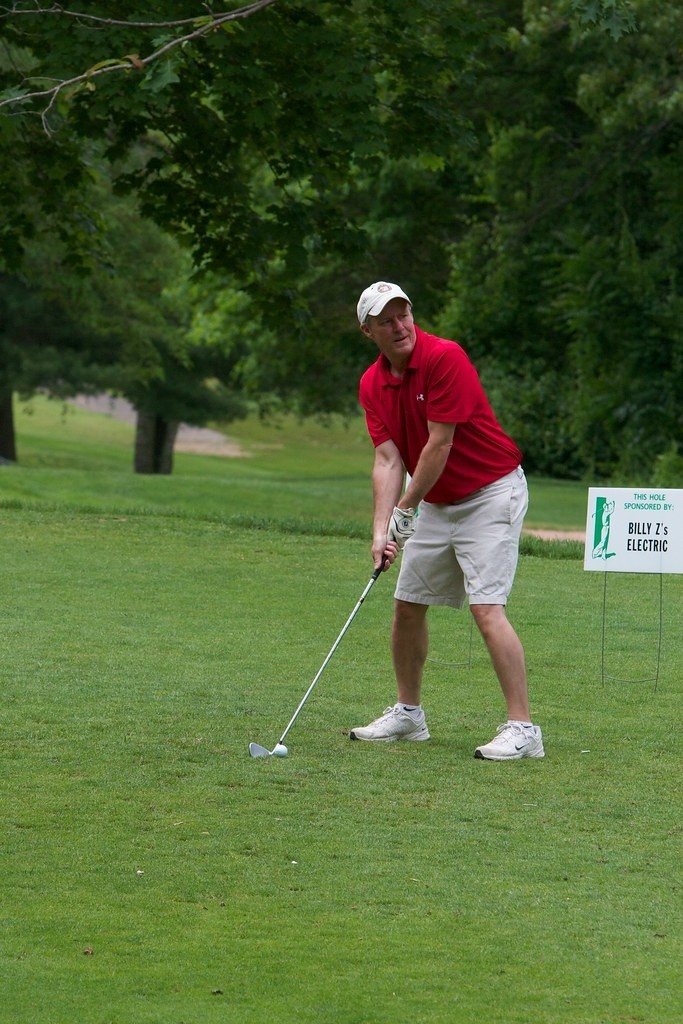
[274,744,288,758]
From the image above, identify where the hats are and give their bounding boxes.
[357,281,412,324]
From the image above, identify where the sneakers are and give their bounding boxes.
[475,723,546,761]
[350,706,430,742]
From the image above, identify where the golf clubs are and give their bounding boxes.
[248,546,392,759]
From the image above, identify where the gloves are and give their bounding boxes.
[386,506,416,548]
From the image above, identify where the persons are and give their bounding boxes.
[349,281,547,760]
